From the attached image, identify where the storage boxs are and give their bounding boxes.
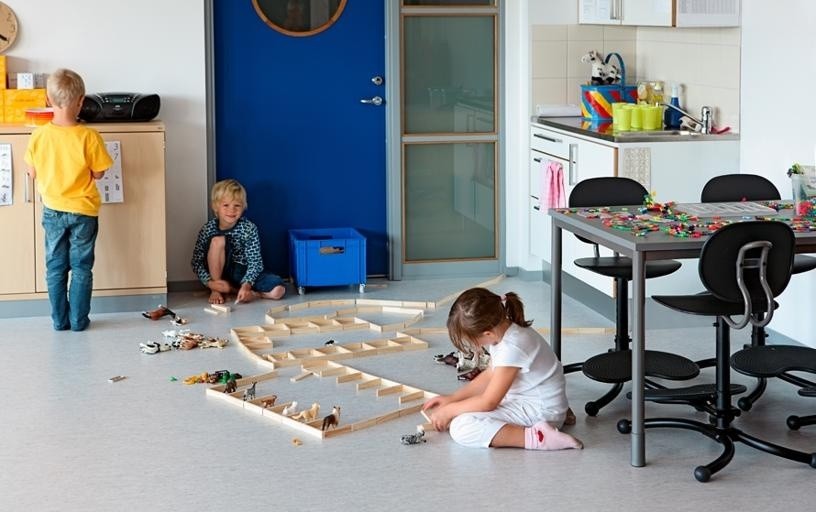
[287,227,367,295]
[3,89,47,125]
[791,166,816,220]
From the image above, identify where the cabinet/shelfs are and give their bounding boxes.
[577,0,742,28]
[452,102,494,235]
[0,120,167,302]
[529,123,741,329]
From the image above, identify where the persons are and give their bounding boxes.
[24,66,114,333]
[189,178,289,305]
[420,285,585,452]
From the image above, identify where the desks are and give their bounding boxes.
[545,198,816,467]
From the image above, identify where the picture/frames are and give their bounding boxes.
[251,0,347,38]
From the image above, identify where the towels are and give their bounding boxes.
[539,158,568,215]
[472,144,490,189]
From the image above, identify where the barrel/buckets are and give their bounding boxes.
[793,165,816,214]
[579,53,638,128]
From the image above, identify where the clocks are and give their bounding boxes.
[0,1,19,53]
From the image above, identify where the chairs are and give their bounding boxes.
[702,174,816,411]
[557,178,700,416]
[619,221,816,483]
[730,343,816,430]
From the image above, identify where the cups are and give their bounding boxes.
[610,101,663,132]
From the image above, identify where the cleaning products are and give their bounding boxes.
[664,85,687,129]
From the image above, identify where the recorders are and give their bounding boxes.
[77,91,161,123]
